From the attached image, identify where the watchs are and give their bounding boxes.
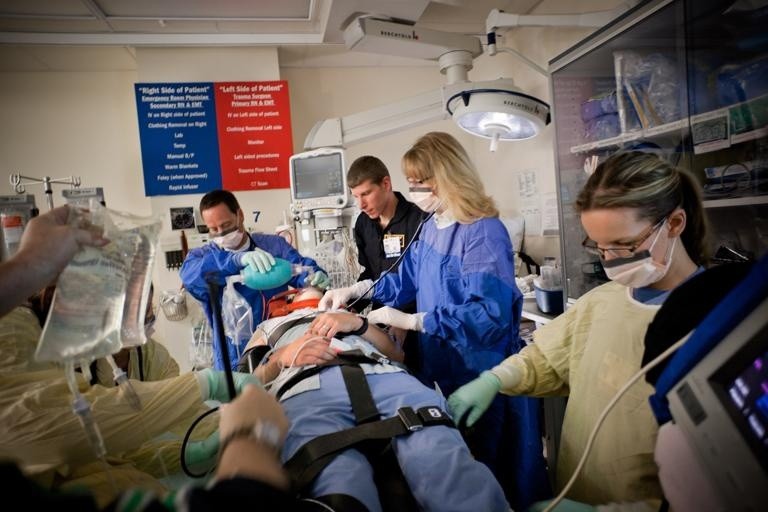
[219,419,286,459]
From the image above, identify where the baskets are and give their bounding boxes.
[160,288,188,322]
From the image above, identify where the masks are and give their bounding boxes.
[409,187,445,212]
[209,210,245,249]
[598,221,676,288]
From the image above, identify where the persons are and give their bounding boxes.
[2,204,291,509]
[179,191,329,368]
[317,133,521,496]
[243,285,511,512]
[447,156,723,511]
[348,156,429,304]
[0,276,263,474]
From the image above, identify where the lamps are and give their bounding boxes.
[340,11,551,153]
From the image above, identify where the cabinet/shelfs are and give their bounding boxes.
[549,0,768,316]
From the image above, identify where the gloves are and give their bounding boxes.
[530,496,594,512]
[447,370,500,427]
[205,369,260,404]
[318,280,374,311]
[367,306,428,333]
[186,430,221,463]
[304,271,330,289]
[241,249,277,275]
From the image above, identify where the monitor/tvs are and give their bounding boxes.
[288,148,358,213]
[664,293,768,511]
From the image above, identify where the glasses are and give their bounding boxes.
[405,174,435,185]
[581,218,665,259]
